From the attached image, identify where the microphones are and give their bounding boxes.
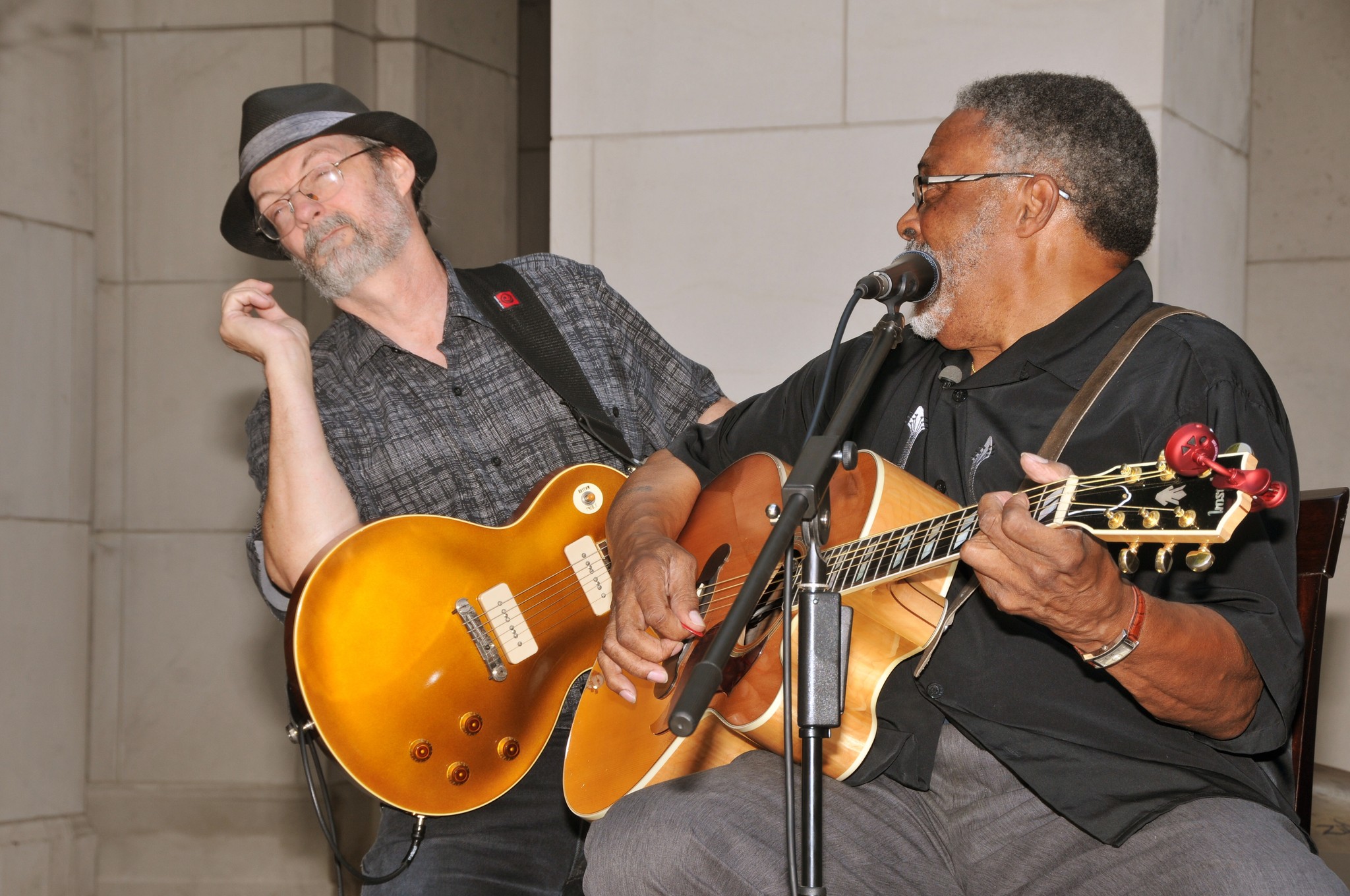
[854,250,942,302]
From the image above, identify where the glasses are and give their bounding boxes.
[912,172,1070,213]
[255,142,390,241]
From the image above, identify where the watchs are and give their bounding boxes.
[1072,581,1147,670]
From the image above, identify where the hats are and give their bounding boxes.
[219,82,437,260]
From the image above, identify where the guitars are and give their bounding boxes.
[283,462,631,818]
[562,420,1289,821]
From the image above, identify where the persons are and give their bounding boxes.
[581,69,1350,896]
[216,81,741,896]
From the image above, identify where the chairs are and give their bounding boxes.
[1285,488,1349,832]
